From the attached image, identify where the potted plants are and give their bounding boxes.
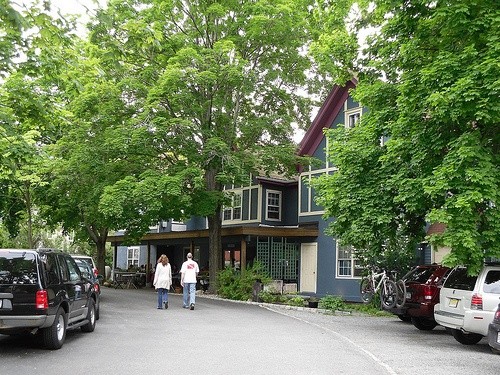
[308,295,319,308]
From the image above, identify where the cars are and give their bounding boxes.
[395,263,451,330]
[67,262,103,298]
[488,298,500,355]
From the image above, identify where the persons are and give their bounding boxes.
[126,264,155,273]
[153,254,172,309]
[179,253,199,310]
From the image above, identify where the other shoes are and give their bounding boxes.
[182,305,189,309]
[164,303,168,309]
[190,303,195,310]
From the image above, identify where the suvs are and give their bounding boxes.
[70,254,98,282]
[433,260,500,346]
[0,247,97,351]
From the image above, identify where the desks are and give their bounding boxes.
[114,271,145,289]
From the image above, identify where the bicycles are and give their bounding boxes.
[359,260,407,311]
